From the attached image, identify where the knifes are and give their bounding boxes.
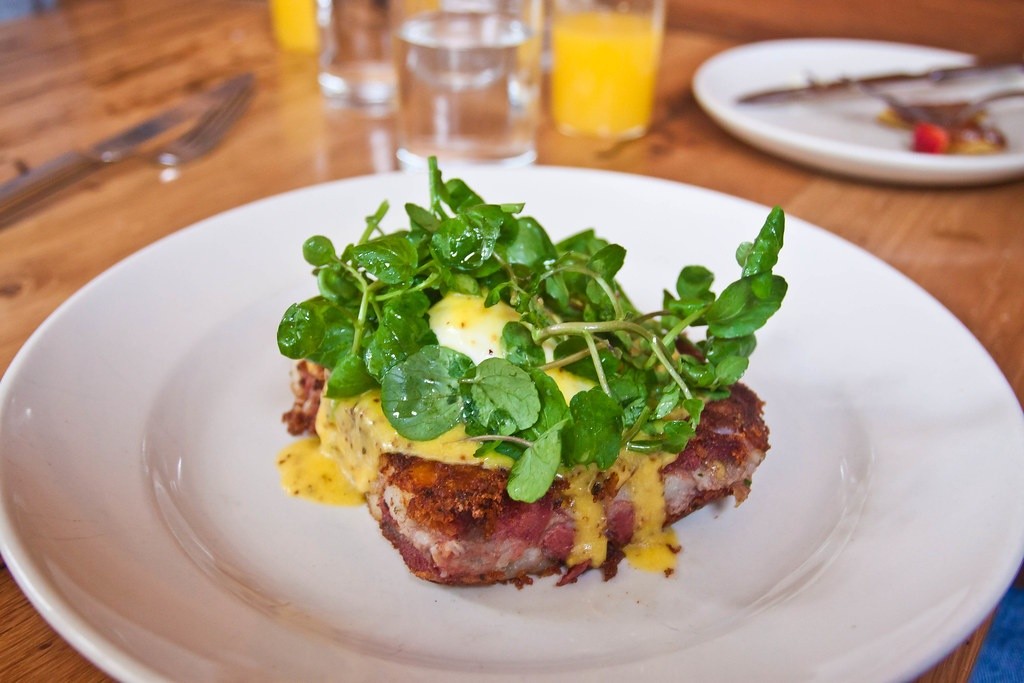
[739,61,1024,107]
[0,105,198,207]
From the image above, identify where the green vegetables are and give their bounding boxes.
[275,157,790,504]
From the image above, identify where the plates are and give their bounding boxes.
[0,166,1024,683]
[692,37,1024,185]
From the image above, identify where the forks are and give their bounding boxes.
[882,85,1024,127]
[0,75,256,230]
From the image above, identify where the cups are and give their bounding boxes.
[548,0,665,137]
[270,0,402,118]
[396,0,544,166]
